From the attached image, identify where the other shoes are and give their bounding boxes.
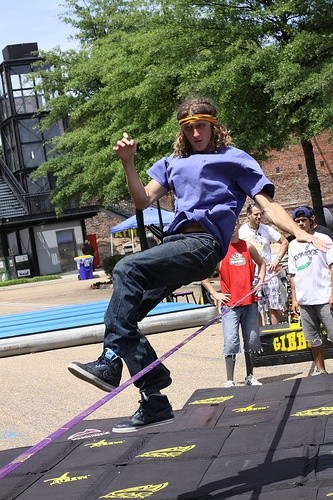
[310,371,328,376]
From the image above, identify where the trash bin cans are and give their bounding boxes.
[74,255,94,280]
[0,257,11,282]
[13,253,36,278]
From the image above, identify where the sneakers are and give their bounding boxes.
[244,375,261,385]
[225,380,234,387]
[111,392,174,434]
[67,349,123,393]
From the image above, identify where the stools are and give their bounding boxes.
[172,291,197,305]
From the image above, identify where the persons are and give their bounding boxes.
[68,97,333,433]
[83,239,96,273]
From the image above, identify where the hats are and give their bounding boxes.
[292,207,311,220]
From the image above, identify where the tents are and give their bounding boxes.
[110,207,175,257]
